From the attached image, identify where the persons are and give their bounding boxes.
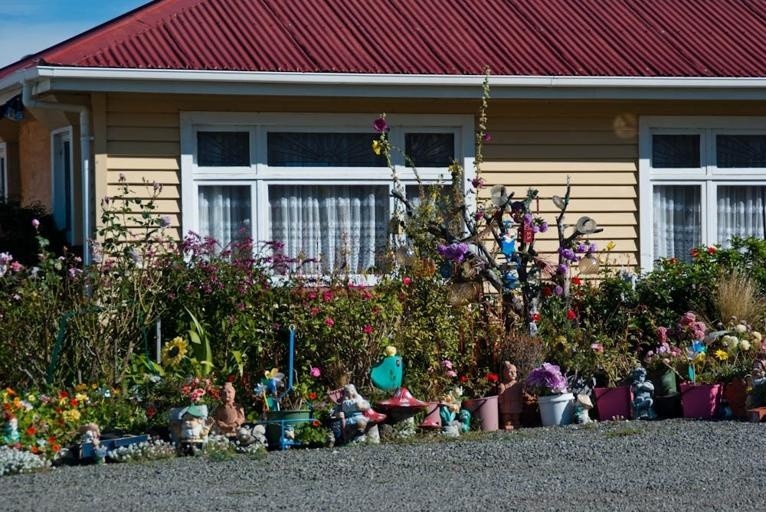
[496,359,536,431]
[632,367,658,420]
[213,381,247,435]
[179,410,205,436]
[342,383,387,445]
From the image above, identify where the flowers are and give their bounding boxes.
[148,312,765,447]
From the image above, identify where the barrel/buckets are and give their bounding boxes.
[537,392,575,428]
[462,395,499,433]
[679,378,724,420]
[594,384,631,420]
[417,401,452,429]
[267,409,311,449]
